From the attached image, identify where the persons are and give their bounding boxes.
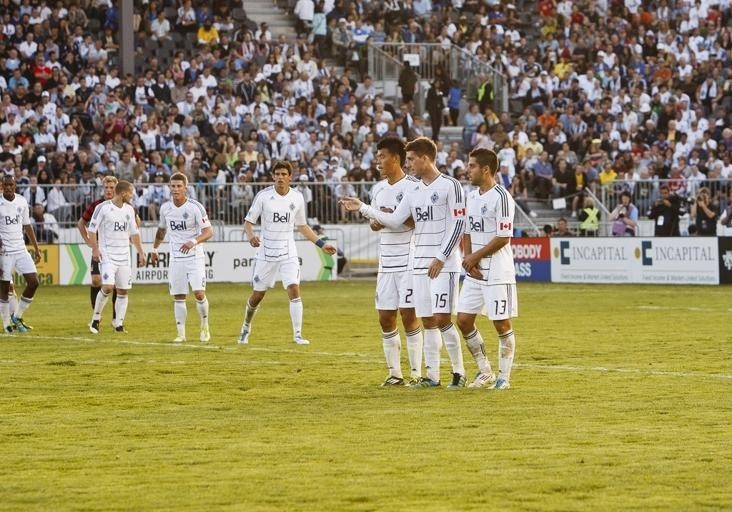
[457,148,518,391]
[238,161,336,346]
[0,0,731,238]
[0,174,41,334]
[369,139,427,389]
[311,224,347,275]
[151,173,213,342]
[77,176,121,319]
[339,137,466,391]
[88,180,147,335]
[8,277,34,331]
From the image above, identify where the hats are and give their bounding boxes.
[699,187,710,195]
[37,156,46,163]
[640,103,651,112]
[42,91,50,98]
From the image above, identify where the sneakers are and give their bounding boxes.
[7,313,33,333]
[200,328,211,342]
[111,319,128,333]
[381,373,509,390]
[292,336,309,345]
[173,336,187,342]
[88,320,100,333]
[238,330,251,343]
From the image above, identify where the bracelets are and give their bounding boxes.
[152,246,160,255]
[190,238,199,248]
[435,252,448,264]
[315,240,326,249]
